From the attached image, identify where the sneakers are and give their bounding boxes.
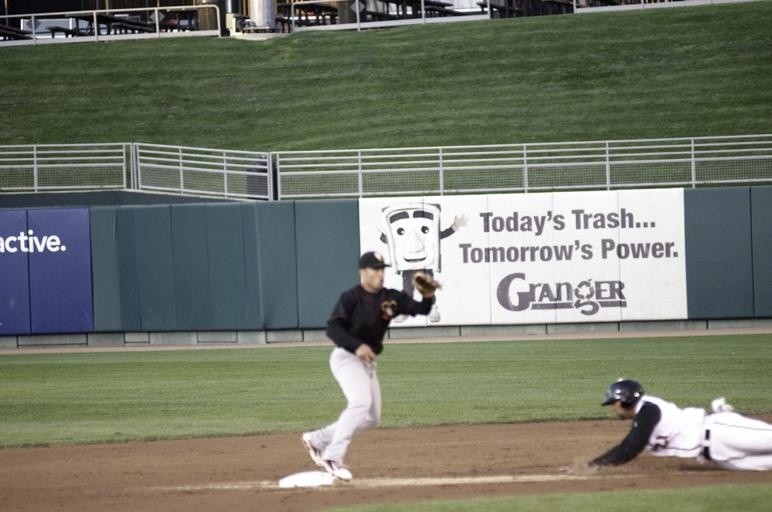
[303,435,353,482]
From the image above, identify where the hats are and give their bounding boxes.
[358,252,392,268]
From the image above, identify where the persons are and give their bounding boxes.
[588,379,772,473]
[302,252,439,480]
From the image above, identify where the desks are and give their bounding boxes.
[78,14,128,34]
[0,22,22,33]
[378,0,453,18]
[283,2,337,26]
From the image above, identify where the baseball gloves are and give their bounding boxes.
[412,272,442,294]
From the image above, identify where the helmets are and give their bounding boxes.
[602,380,645,408]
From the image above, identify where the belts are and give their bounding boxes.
[704,429,709,459]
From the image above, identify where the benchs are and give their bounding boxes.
[47,26,82,37]
[0,29,34,39]
[110,22,155,34]
[477,2,512,14]
[425,5,465,17]
